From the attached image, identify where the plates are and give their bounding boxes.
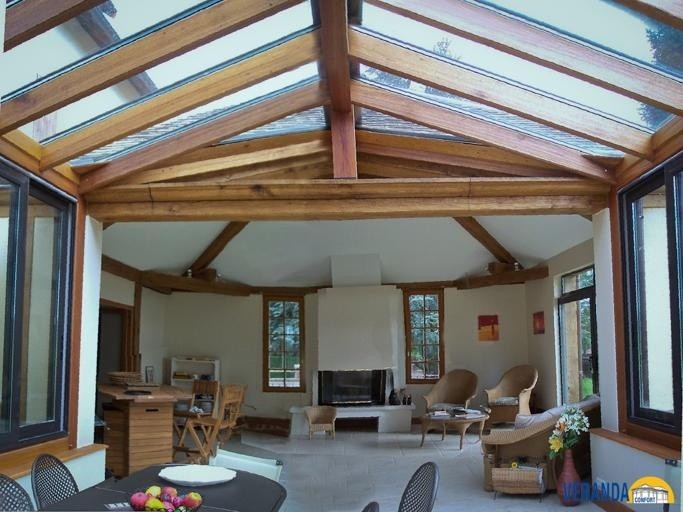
[157,464,237,488]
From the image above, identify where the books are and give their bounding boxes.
[425,407,486,420]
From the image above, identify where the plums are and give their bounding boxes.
[173,497,183,507]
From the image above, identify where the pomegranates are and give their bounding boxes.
[130,492,147,509]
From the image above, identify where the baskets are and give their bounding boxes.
[125,486,206,512]
[106,371,139,386]
[491,458,548,494]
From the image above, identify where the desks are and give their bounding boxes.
[289,404,416,436]
[38,463,287,512]
[97,384,193,478]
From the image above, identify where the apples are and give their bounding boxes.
[145,485,161,500]
[183,492,201,508]
[161,486,178,501]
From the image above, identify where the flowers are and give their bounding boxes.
[548,405,591,461]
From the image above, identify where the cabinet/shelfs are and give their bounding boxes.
[170,354,220,427]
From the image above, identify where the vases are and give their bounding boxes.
[557,449,582,506]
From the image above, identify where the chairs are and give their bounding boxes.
[173,381,245,464]
[483,364,538,425]
[209,447,284,483]
[0,473,35,512]
[398,461,440,512]
[31,454,80,512]
[304,406,337,441]
[480,395,600,490]
[422,369,479,409]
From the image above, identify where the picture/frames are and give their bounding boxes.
[146,366,154,383]
